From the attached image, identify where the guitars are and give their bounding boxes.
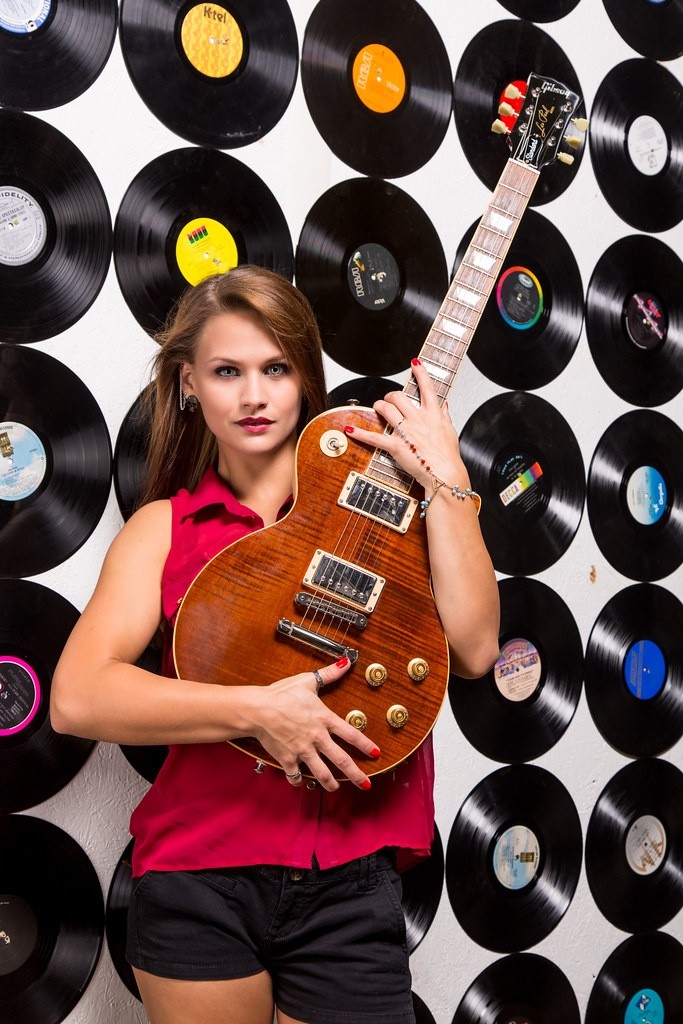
[172,71,594,784]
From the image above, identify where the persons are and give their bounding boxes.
[48,263,503,1024]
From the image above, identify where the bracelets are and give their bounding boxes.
[397,427,482,518]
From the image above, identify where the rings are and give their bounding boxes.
[285,769,303,780]
[312,669,326,688]
[393,416,405,430]
